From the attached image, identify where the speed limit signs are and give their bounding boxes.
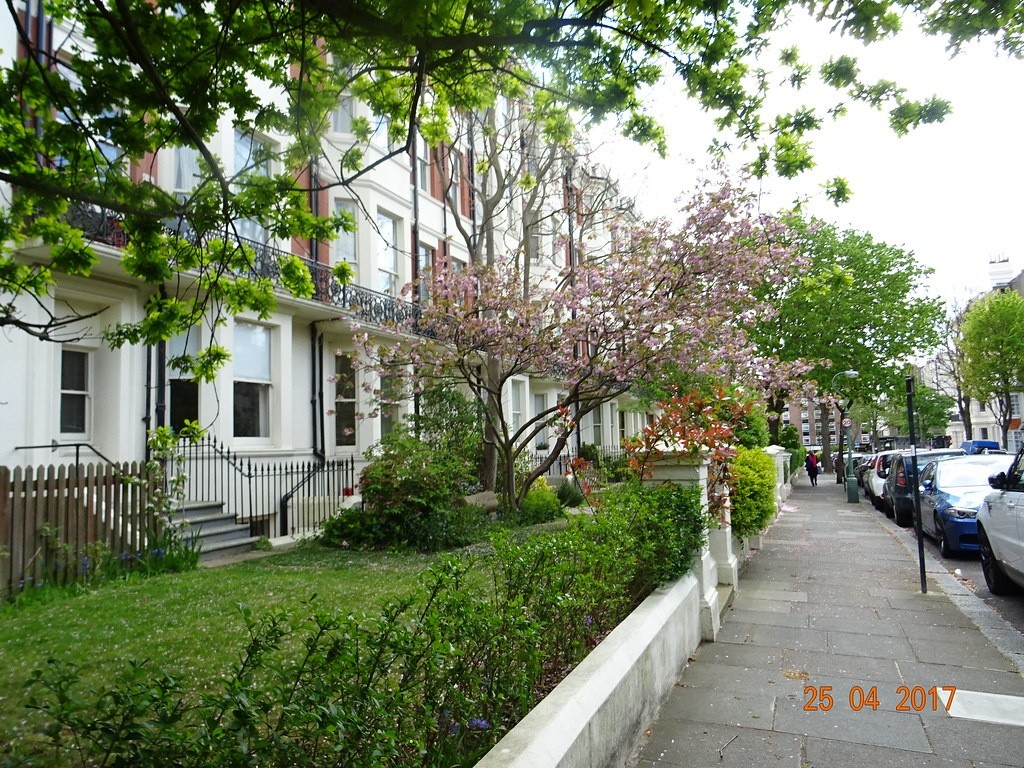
[841,417,852,427]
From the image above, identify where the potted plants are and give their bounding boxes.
[536,442,550,455]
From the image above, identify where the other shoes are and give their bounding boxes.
[813,483,814,487]
[815,481,817,485]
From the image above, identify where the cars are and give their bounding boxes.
[831,436,1024,596]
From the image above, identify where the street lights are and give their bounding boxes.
[831,370,861,505]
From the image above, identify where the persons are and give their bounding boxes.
[804,448,819,487]
